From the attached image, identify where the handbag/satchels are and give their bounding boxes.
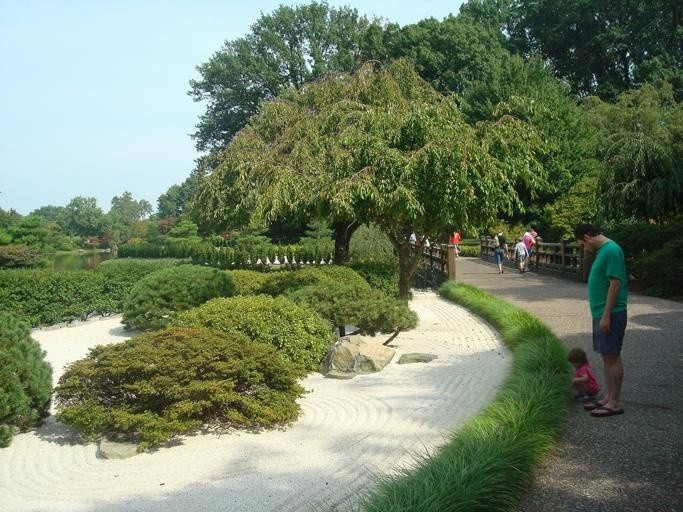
[495,234,500,247]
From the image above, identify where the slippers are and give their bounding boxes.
[584,400,603,411]
[591,407,624,417]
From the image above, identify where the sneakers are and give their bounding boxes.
[519,267,530,272]
[499,270,504,275]
[574,394,592,402]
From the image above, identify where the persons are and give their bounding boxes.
[572,222,629,418]
[493,227,538,275]
[448,230,461,257]
[408,231,416,249]
[567,347,601,402]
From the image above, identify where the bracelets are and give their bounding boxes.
[600,316,611,322]
